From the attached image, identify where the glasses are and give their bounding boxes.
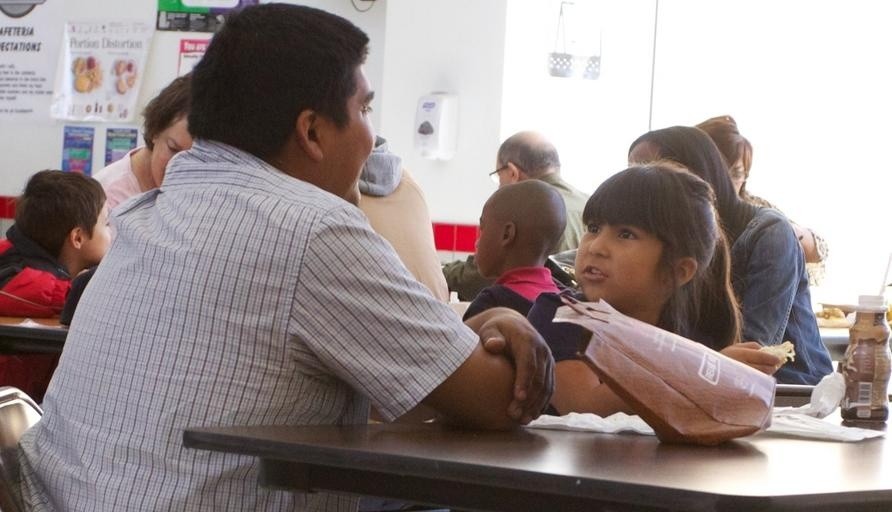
[728,172,751,183]
[484,166,507,181]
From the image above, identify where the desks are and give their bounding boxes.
[182,415,890,512]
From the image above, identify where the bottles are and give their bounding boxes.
[835,295,892,428]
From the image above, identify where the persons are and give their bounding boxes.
[0,168,113,317]
[625,115,835,391]
[696,115,829,285]
[463,180,568,320]
[440,132,595,300]
[344,133,451,307]
[527,160,794,419]
[0,4,555,512]
[91,73,194,213]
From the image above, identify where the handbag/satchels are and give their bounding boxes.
[551,295,776,448]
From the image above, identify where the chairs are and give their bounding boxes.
[0,386,61,512]
